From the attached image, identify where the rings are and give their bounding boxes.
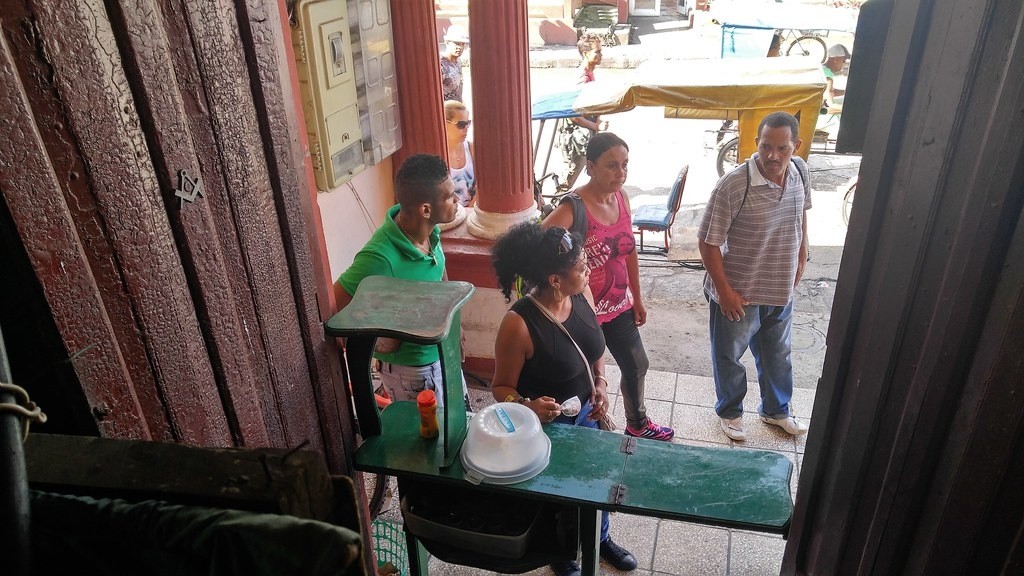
[596,402,603,405]
[548,410,553,417]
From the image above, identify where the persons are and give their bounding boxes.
[817,43,852,139]
[552,32,610,206]
[489,214,639,576]
[531,131,675,442]
[439,24,469,106]
[331,152,475,413]
[696,111,812,442]
[443,99,478,208]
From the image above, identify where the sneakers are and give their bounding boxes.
[598,534,636,572]
[551,559,583,576]
[760,412,808,435]
[624,417,675,442]
[720,417,747,441]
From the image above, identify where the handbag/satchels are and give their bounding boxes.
[595,413,617,433]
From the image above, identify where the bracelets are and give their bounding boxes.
[594,375,609,388]
[503,393,530,405]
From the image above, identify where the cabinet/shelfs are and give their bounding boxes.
[322,275,792,576]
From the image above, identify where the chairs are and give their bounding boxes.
[632,163,689,253]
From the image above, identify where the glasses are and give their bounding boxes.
[555,225,574,258]
[446,119,472,129]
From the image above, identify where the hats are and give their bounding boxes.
[443,25,469,44]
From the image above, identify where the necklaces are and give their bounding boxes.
[449,142,464,162]
[398,210,438,266]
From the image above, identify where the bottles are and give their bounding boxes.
[417,390,439,438]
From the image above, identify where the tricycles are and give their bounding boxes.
[527,56,827,269]
[709,0,859,180]
